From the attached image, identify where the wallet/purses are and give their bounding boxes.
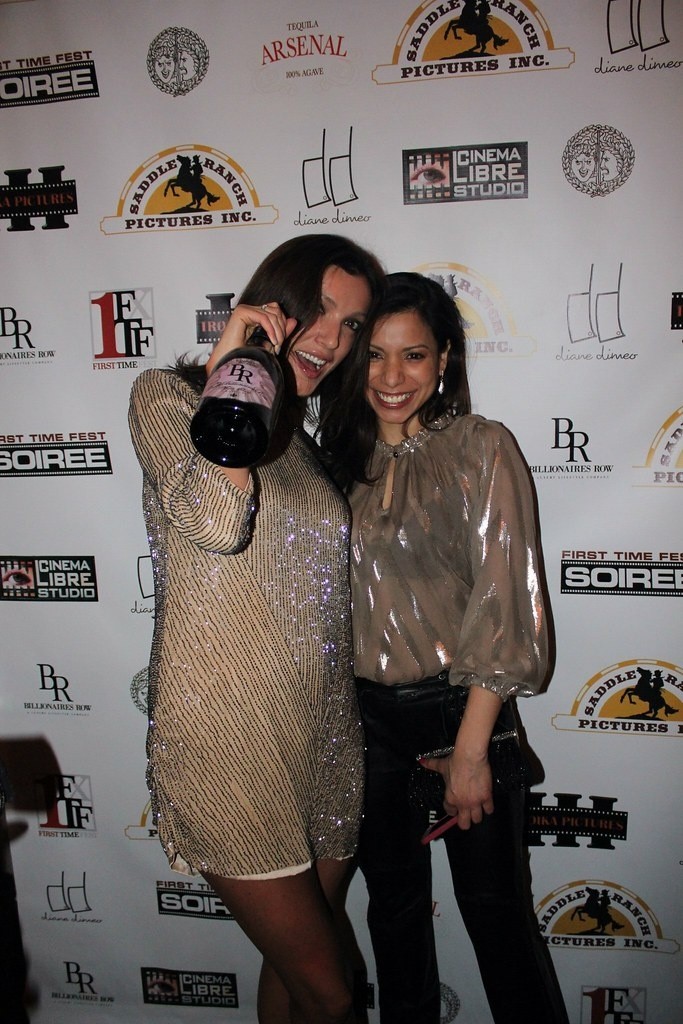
[405,725,546,811]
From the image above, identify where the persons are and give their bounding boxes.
[127,232,384,1024]
[310,271,573,1024]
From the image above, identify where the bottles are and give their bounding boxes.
[190,327,282,468]
